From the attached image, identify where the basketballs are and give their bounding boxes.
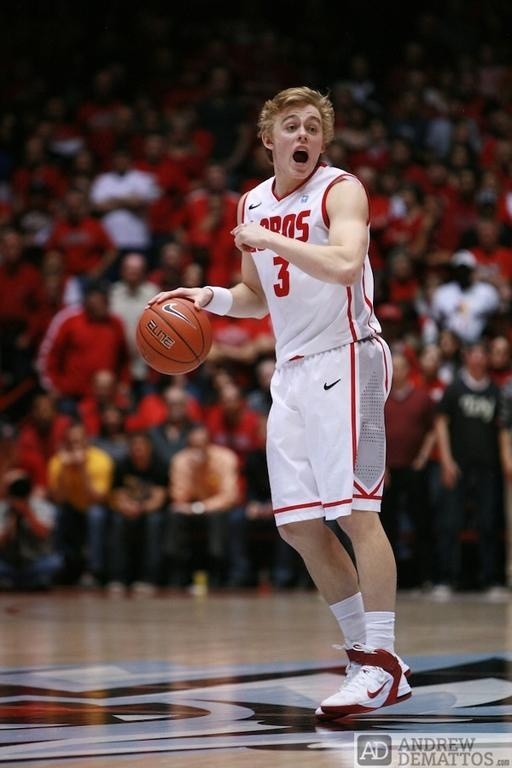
[135,297,210,374]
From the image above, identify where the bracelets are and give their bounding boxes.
[201,285,233,318]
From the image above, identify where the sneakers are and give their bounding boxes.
[408,578,510,607]
[314,645,415,724]
[74,569,298,600]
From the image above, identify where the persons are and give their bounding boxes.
[0,15,512,604]
[142,83,416,723]
